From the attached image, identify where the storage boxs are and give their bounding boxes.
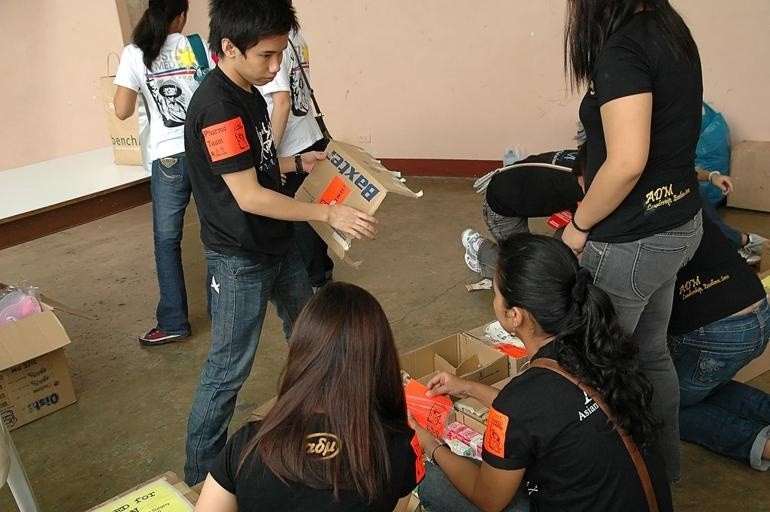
[293,133,423,268]
[0,278,99,432]
[79,470,206,510]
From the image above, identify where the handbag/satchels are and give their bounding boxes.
[473,168,499,197]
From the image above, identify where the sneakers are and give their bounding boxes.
[459,225,484,275]
[137,323,194,349]
[736,231,770,266]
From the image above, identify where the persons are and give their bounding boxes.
[112,0,218,344]
[411,231,674,511]
[255,25,332,193]
[558,0,704,505]
[458,148,586,282]
[184,1,381,484]
[667,209,770,473]
[693,160,770,269]
[192,280,428,512]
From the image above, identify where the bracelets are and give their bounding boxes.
[707,170,720,180]
[431,438,448,465]
[294,152,305,174]
[571,216,590,233]
